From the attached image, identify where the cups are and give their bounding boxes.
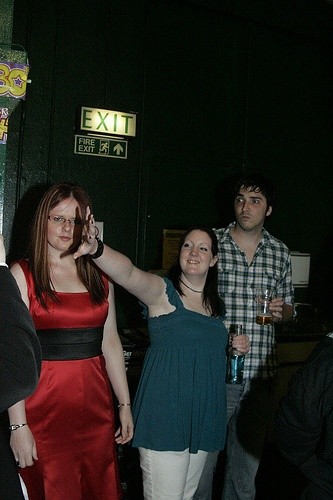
[256,288,275,324]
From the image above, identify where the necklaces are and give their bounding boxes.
[179,277,203,293]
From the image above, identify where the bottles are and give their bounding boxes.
[226,324,245,385]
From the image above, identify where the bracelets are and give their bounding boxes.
[87,237,104,259]
[8,423,28,431]
[117,403,132,408]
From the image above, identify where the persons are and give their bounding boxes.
[273,331,333,500]
[72,205,252,500]
[7,180,135,500]
[213,172,298,500]
[0,237,43,500]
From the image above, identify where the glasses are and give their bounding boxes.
[48,215,86,226]
